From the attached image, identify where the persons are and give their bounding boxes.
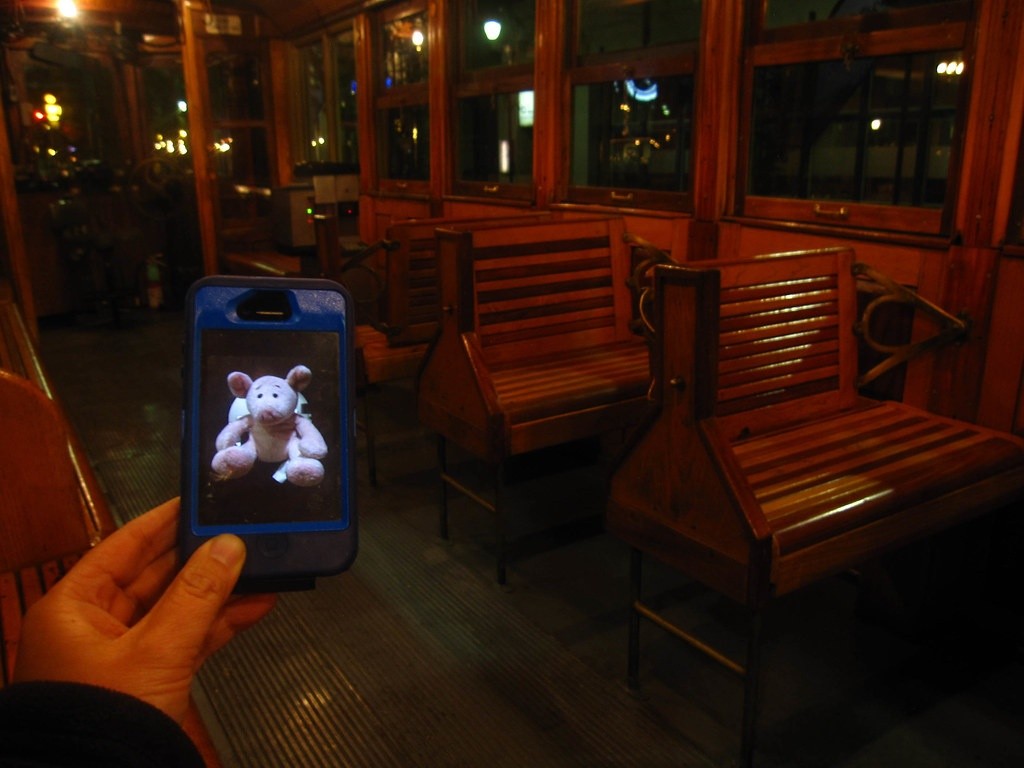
[0,496,279,768]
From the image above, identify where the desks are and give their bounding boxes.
[217,248,304,278]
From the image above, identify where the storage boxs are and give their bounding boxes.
[270,183,319,250]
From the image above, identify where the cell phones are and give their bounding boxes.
[179,283,358,580]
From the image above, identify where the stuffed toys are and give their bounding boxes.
[212,366,327,488]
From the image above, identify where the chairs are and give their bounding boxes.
[0,368,93,688]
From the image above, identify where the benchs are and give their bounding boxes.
[352,209,1024,768]
